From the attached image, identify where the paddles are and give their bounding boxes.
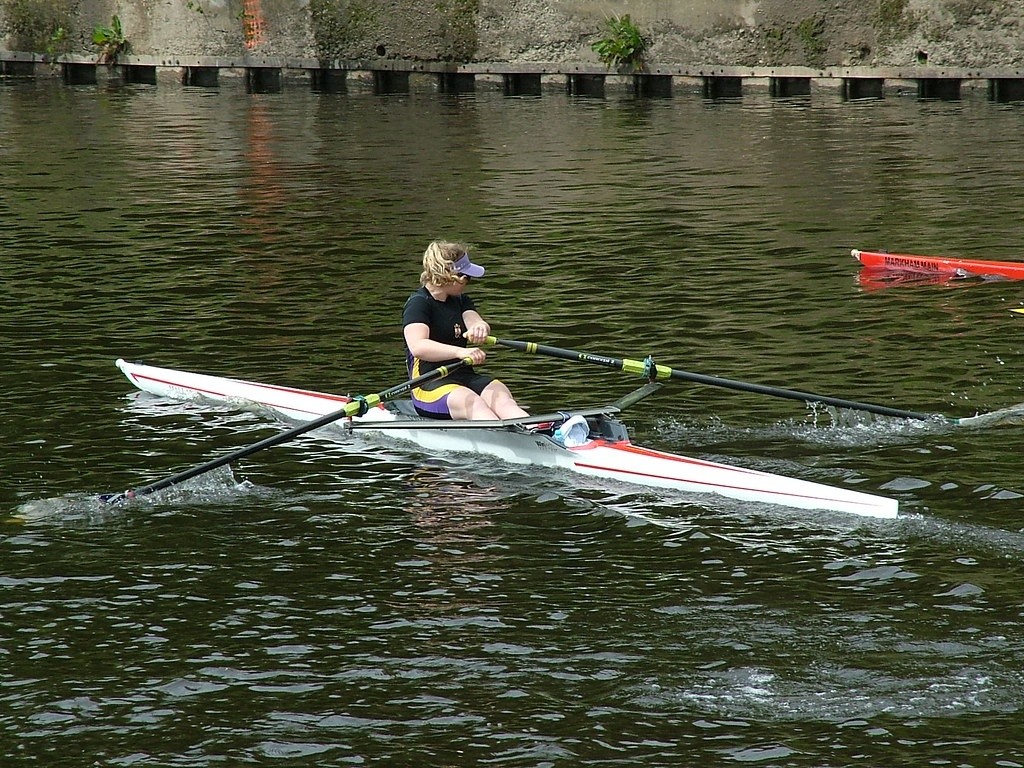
[92,356,474,510]
[466,332,1024,429]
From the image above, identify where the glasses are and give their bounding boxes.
[454,272,471,281]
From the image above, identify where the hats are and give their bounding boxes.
[446,252,485,278]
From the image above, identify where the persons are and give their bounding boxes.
[401,240,554,432]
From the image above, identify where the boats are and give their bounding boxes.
[849,248,1024,279]
[113,353,899,521]
